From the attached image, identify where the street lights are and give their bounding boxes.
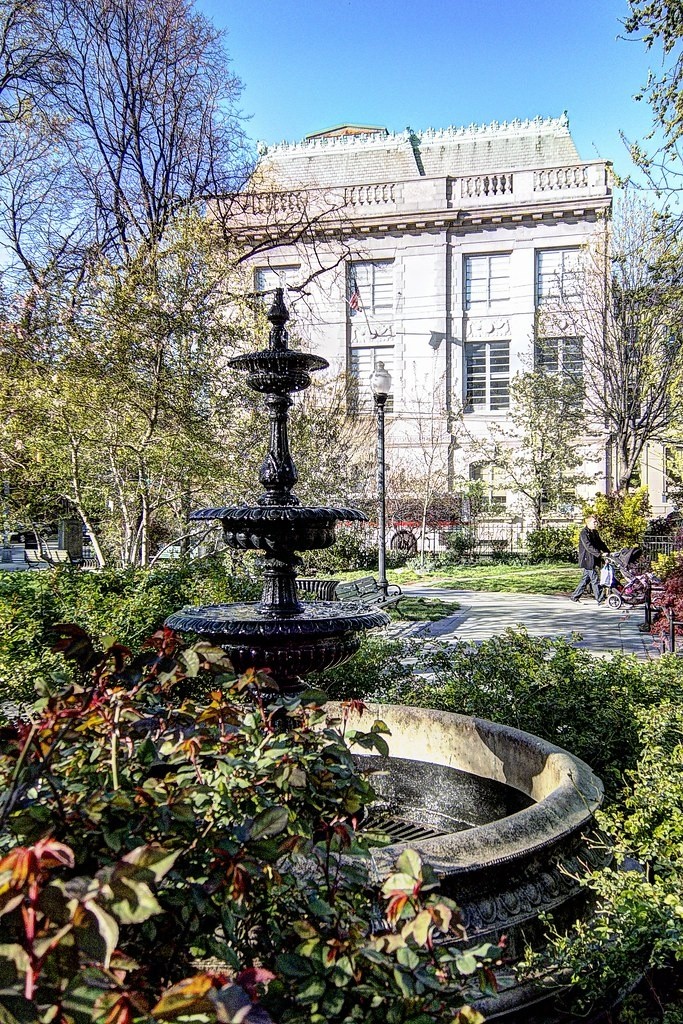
[369,361,392,597]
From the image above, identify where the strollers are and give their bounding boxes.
[603,547,663,609]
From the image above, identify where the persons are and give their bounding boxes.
[569,516,610,608]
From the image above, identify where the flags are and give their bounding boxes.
[349,278,362,312]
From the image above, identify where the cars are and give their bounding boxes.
[82,521,103,545]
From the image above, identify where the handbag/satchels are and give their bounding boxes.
[600,563,613,587]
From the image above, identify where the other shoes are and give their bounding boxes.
[599,604,609,608]
[569,599,584,605]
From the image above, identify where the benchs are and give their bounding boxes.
[335,577,405,619]
[639,581,683,654]
[22,549,84,567]
